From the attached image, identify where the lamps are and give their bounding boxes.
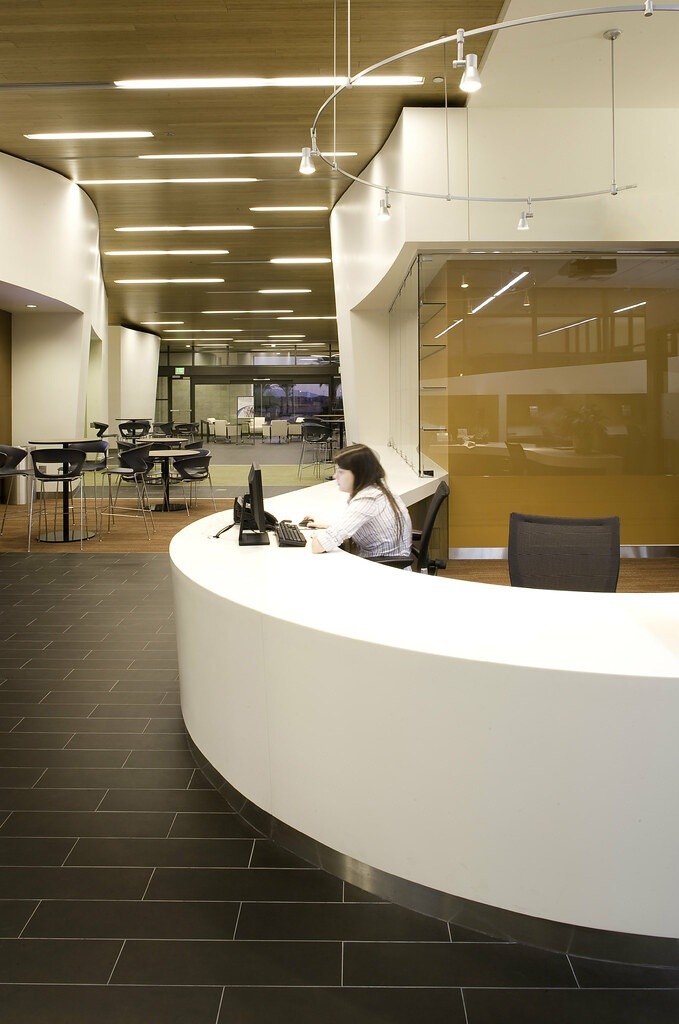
[522,284,531,307]
[450,27,482,93]
[298,127,317,175]
[374,186,394,222]
[517,198,535,230]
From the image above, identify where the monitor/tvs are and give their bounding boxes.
[239,461,270,545]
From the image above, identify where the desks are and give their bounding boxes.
[28,436,102,543]
[115,418,153,445]
[143,449,200,512]
[135,438,189,445]
[314,414,344,461]
[320,420,346,451]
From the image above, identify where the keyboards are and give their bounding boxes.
[275,523,307,547]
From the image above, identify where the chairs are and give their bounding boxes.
[508,512,621,593]
[364,480,450,573]
[0,415,348,554]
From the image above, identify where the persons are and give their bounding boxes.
[306,443,413,574]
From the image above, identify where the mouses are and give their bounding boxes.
[299,520,316,529]
